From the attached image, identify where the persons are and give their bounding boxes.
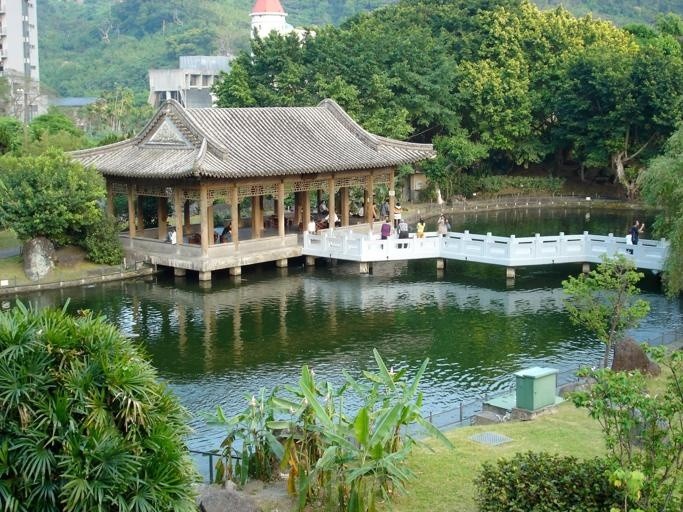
[379,219,390,249]
[372,198,402,222]
[396,218,408,248]
[416,218,425,237]
[630,219,644,254]
[349,202,364,218]
[436,214,450,236]
[625,229,632,252]
[318,200,327,212]
